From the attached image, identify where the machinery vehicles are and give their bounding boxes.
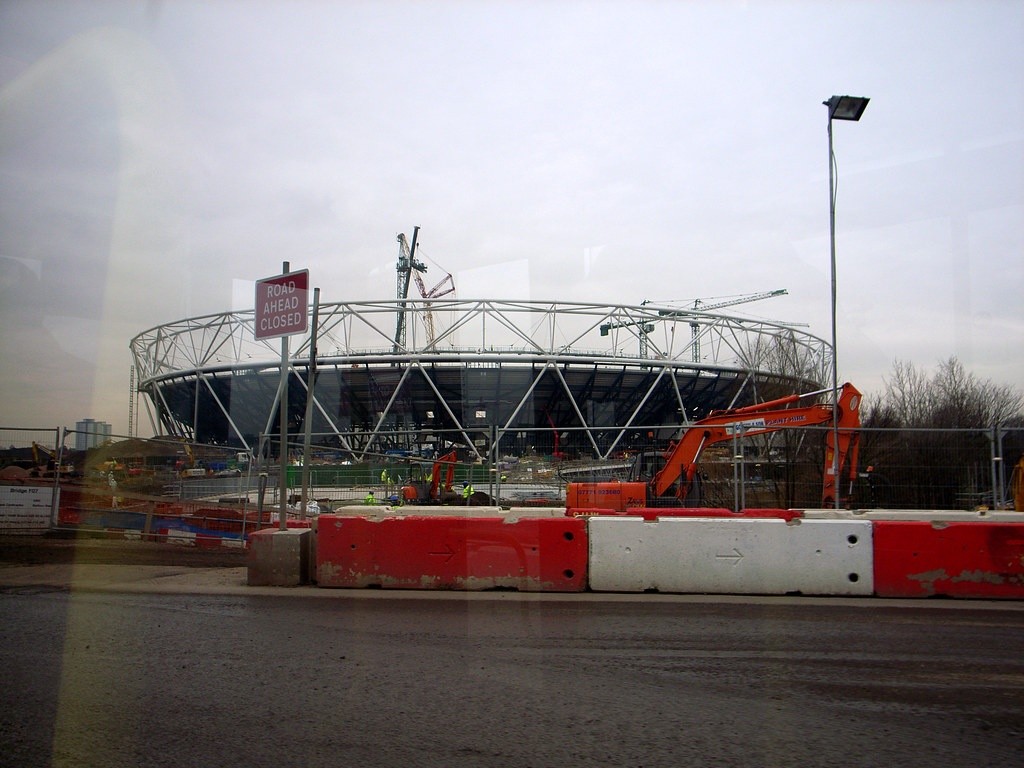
[560,382,863,510]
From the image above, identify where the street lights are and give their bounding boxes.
[822,92,871,506]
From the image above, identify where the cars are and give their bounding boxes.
[509,491,562,508]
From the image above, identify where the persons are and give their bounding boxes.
[380,469,387,485]
[108,471,113,487]
[111,479,118,492]
[111,495,123,508]
[110,457,117,470]
[365,491,376,506]
[461,482,474,505]
[388,475,394,488]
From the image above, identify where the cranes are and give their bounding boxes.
[395,226,456,367]
[658,298,809,373]
[600,288,788,370]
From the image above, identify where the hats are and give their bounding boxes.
[110,471,113,473]
[462,481,470,484]
[112,479,115,481]
[390,496,399,500]
[369,491,374,494]
